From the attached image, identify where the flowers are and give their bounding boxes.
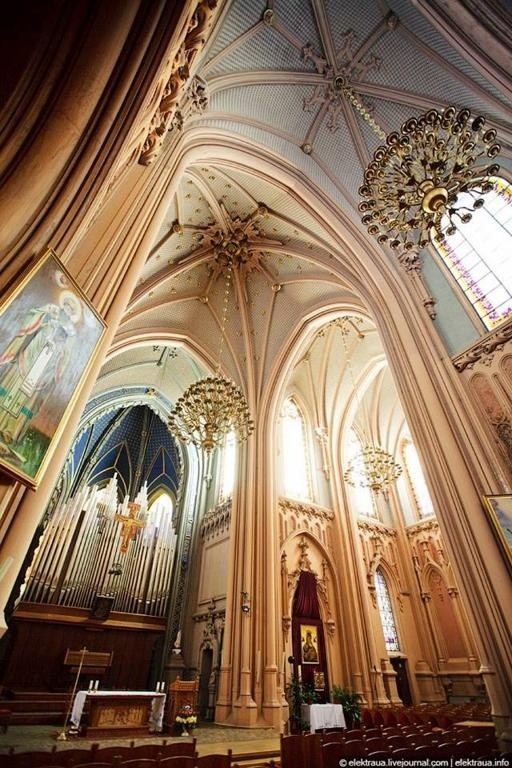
[174,705,200,729]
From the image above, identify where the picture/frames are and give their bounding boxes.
[0,245,110,493]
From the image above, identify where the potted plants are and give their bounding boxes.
[332,687,364,730]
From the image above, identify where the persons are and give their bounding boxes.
[0,297,79,454]
[302,633,318,661]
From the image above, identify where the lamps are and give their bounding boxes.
[165,245,256,490]
[325,71,503,255]
[338,323,403,496]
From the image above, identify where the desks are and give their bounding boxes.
[301,703,346,734]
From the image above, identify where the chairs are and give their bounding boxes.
[1,720,504,768]
